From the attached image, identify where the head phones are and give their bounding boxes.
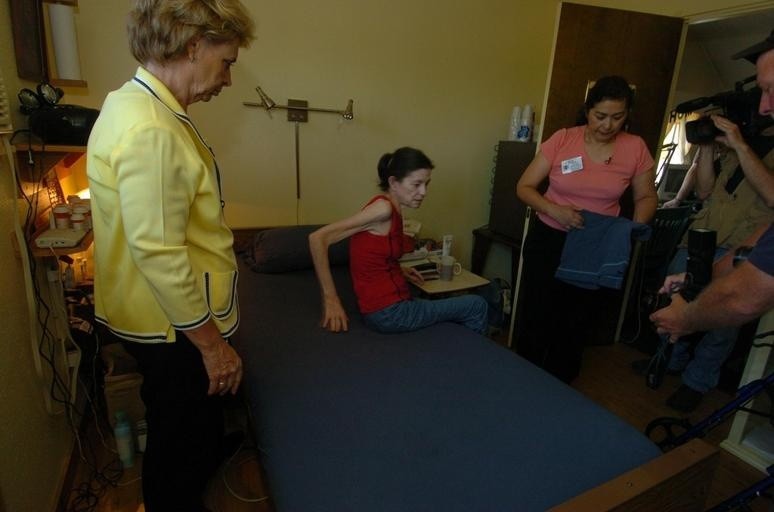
[17,82,64,115]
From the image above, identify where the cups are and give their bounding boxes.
[439,256,462,282]
[53,194,91,232]
[508,101,533,141]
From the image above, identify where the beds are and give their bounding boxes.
[229,225,716,512]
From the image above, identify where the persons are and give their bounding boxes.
[306,145,492,338]
[512,73,658,386]
[85,1,256,512]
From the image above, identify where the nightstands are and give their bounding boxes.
[407,264,490,300]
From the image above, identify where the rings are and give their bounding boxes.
[218,382,226,385]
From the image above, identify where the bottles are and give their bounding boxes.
[113,410,136,470]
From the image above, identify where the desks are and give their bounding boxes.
[471,229,520,315]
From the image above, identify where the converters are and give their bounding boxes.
[62,288,84,300]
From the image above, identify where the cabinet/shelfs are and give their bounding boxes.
[15,138,94,257]
[470,140,536,242]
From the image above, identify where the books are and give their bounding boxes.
[421,272,441,282]
[410,262,438,273]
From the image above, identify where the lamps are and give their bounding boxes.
[253,84,276,109]
[342,98,357,122]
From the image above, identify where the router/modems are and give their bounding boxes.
[35,211,88,249]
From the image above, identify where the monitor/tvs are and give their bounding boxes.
[658,164,691,202]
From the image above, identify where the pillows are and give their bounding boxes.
[245,228,348,275]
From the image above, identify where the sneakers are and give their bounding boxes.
[664,384,702,414]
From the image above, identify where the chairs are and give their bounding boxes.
[649,204,693,268]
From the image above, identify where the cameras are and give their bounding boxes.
[642,228,721,313]
[732,245,754,265]
